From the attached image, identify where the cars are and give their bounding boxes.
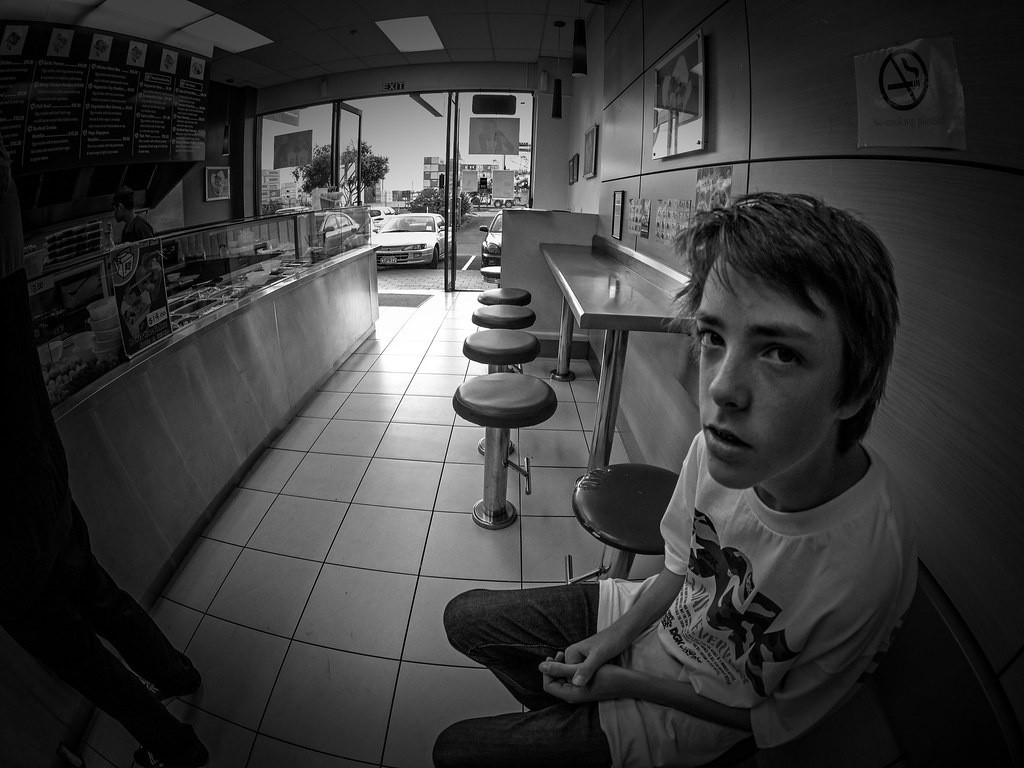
[479,210,503,269]
[314,213,362,249]
[366,206,396,231]
[369,213,452,269]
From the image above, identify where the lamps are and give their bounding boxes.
[221,79,230,155]
[572,0,588,78]
[551,21,564,119]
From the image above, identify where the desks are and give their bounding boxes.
[539,241,696,471]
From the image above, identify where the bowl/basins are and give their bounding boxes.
[167,272,181,282]
[38,296,123,367]
[246,271,270,285]
[260,260,282,272]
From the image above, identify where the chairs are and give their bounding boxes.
[425,222,434,231]
[712,562,1023,768]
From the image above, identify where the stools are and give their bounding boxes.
[471,303,537,375]
[480,266,501,288]
[452,372,558,526]
[556,460,681,583]
[463,329,541,455]
[476,286,532,307]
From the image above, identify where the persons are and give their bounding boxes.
[111,188,155,335]
[433,193,913,767]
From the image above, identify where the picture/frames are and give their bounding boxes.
[611,189,624,239]
[569,153,578,184]
[205,167,231,201]
[584,122,597,179]
